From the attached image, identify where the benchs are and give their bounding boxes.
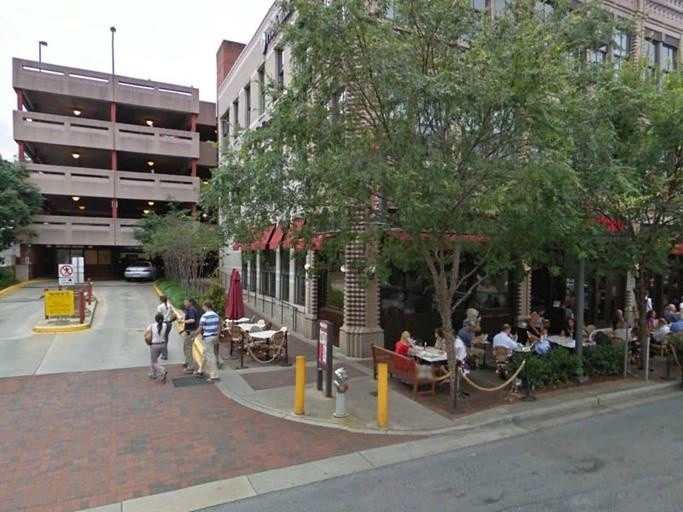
[370,341,435,401]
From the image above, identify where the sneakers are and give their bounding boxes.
[182,364,220,382]
[148,357,168,380]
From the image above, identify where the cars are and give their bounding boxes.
[123,260,158,280]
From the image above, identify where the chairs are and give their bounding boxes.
[228,313,288,363]
[494,346,508,373]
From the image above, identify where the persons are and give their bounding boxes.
[190,297,222,383]
[142,311,167,385]
[392,289,683,388]
[175,296,201,373]
[155,295,174,361]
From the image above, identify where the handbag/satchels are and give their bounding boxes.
[144,331,151,345]
[171,310,176,322]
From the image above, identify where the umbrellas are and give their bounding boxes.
[221,268,246,340]
